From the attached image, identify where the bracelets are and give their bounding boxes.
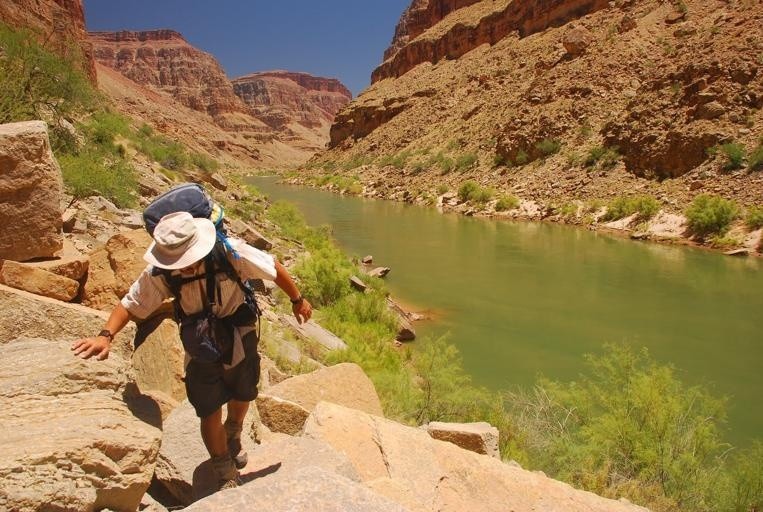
[98,330,114,340]
[290,295,304,305]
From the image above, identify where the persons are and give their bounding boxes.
[72,212,313,491]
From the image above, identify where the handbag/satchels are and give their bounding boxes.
[179,310,233,359]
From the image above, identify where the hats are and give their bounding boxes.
[143,212,216,269]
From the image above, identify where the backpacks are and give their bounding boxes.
[142,185,258,305]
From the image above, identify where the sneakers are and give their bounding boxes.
[212,438,248,491]
